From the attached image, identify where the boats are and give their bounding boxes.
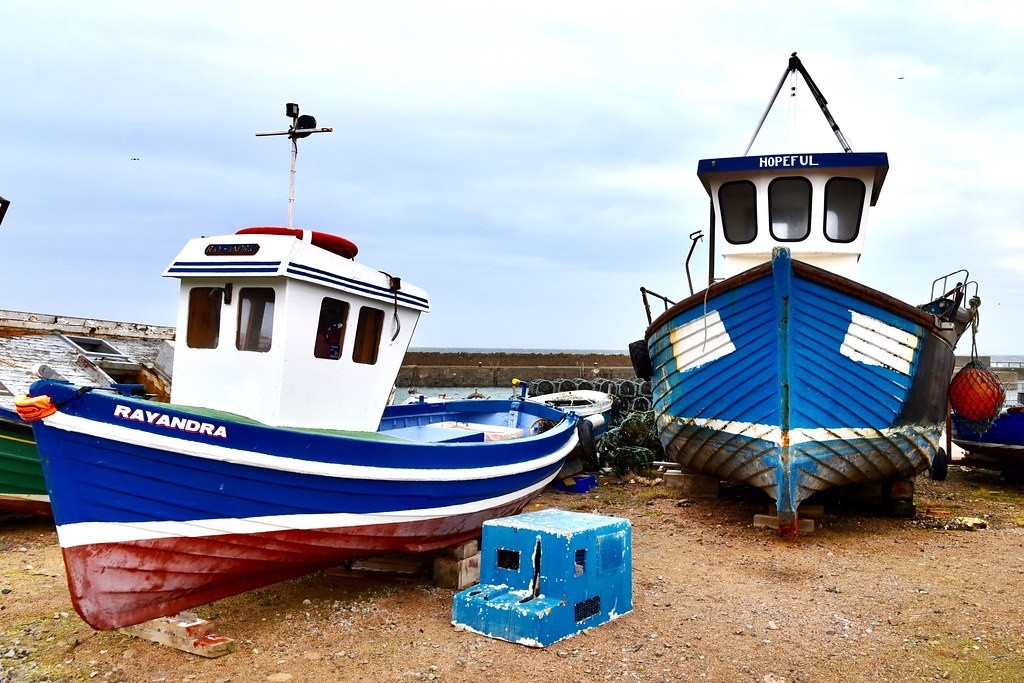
[0,308,273,521]
[511,378,613,438]
[11,101,580,631]
[630,51,979,535]
[943,389,1024,473]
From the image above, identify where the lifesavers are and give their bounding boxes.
[629,340,653,379]
[579,419,600,472]
[235,226,358,259]
